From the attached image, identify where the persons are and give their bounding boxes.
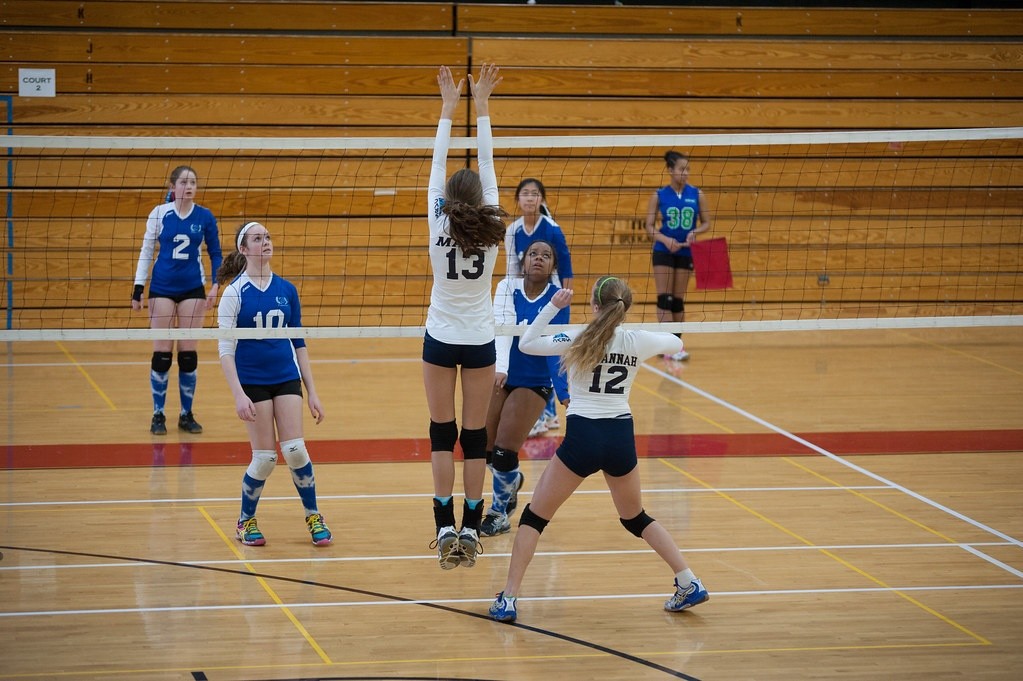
[504,178,573,438]
[422,63,504,570]
[216,221,333,546]
[489,275,710,623]
[644,151,711,361]
[479,241,571,538]
[132,164,223,435]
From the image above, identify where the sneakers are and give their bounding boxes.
[491,621,517,644]
[304,513,333,545]
[659,346,689,360]
[503,472,523,516]
[664,578,709,611]
[458,526,483,568]
[479,507,511,536]
[428,525,458,571]
[489,590,518,620]
[665,611,712,641]
[178,412,202,433]
[545,418,559,429]
[150,412,166,434]
[527,418,548,436]
[235,516,265,545]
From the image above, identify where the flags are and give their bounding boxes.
[690,237,734,290]
